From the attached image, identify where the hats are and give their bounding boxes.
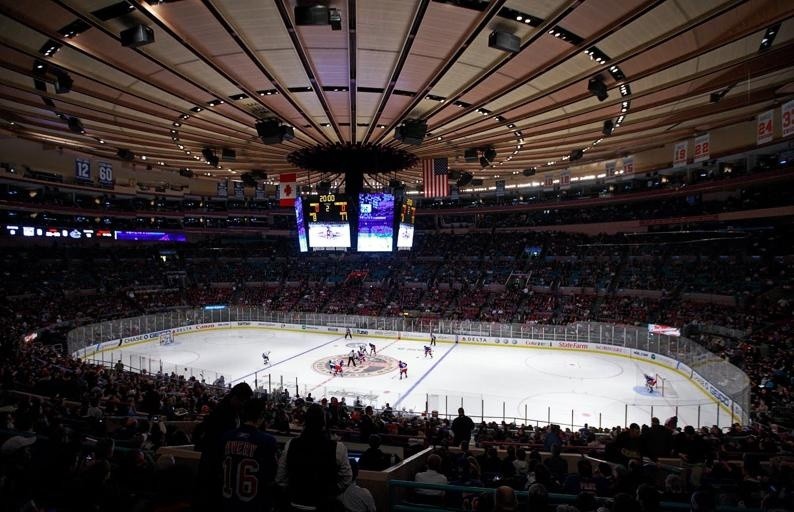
[631,423,640,433]
[668,416,677,429]
[516,448,526,457]
[427,454,441,466]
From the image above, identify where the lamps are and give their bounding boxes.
[395,118,429,146]
[487,29,521,52]
[202,144,268,188]
[52,70,74,93]
[256,116,293,145]
[121,24,155,50]
[293,0,343,32]
[448,146,497,186]
[588,76,610,101]
